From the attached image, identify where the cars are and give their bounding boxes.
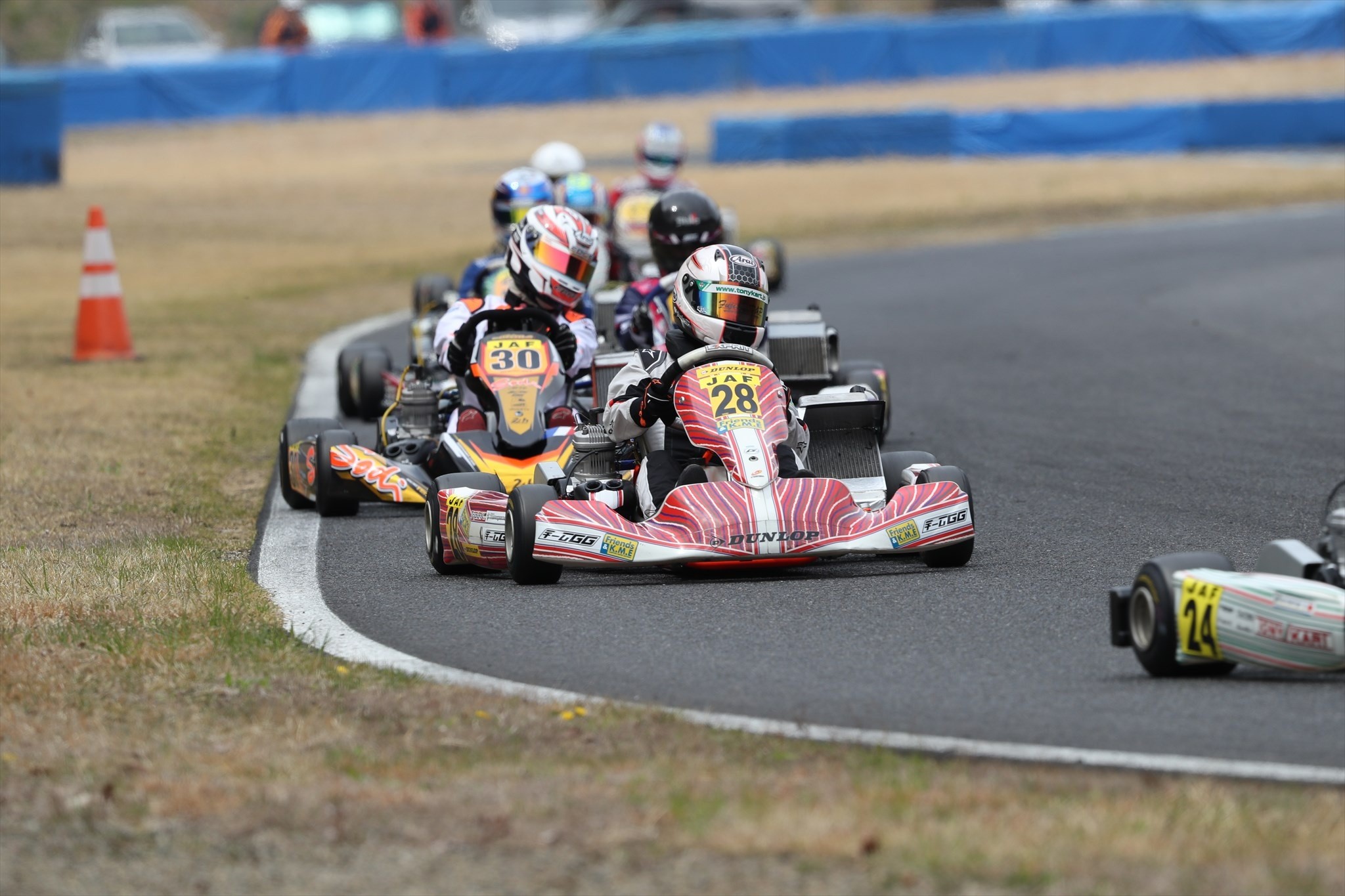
[71,4,223,65]
[248,0,436,49]
[449,0,815,49]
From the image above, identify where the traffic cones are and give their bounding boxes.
[65,204,147,362]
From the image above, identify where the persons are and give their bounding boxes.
[459,141,616,322]
[603,243,816,521]
[433,205,599,433]
[604,121,731,281]
[614,186,729,355]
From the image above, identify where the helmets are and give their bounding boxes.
[634,125,688,186]
[553,172,612,232]
[505,203,599,312]
[530,142,586,179]
[648,192,725,276]
[672,243,770,349]
[613,194,657,261]
[491,165,555,245]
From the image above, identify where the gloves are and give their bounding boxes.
[448,331,470,377]
[781,385,792,424]
[630,378,675,428]
[630,301,651,335]
[547,323,577,371]
